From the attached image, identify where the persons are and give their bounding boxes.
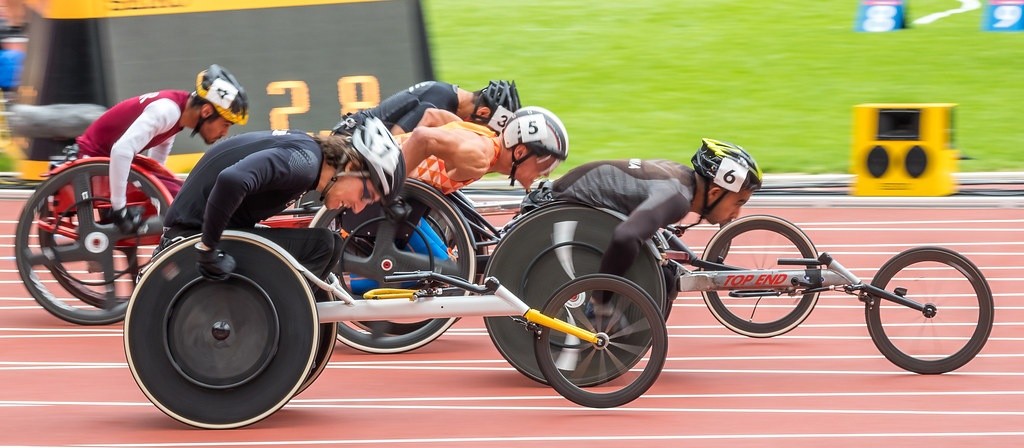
[0,37,28,128]
[152,109,406,294]
[77,64,249,236]
[379,106,570,225]
[522,137,764,317]
[333,79,520,137]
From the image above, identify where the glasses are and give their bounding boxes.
[535,151,559,174]
[361,178,371,203]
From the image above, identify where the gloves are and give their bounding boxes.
[194,243,232,282]
[583,302,613,318]
[382,193,413,224]
[113,207,138,234]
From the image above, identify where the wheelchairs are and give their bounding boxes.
[120,178,996,432]
[14,146,318,328]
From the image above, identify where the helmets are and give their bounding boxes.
[353,116,406,205]
[195,64,249,125]
[503,106,568,160]
[691,138,763,191]
[484,80,521,113]
